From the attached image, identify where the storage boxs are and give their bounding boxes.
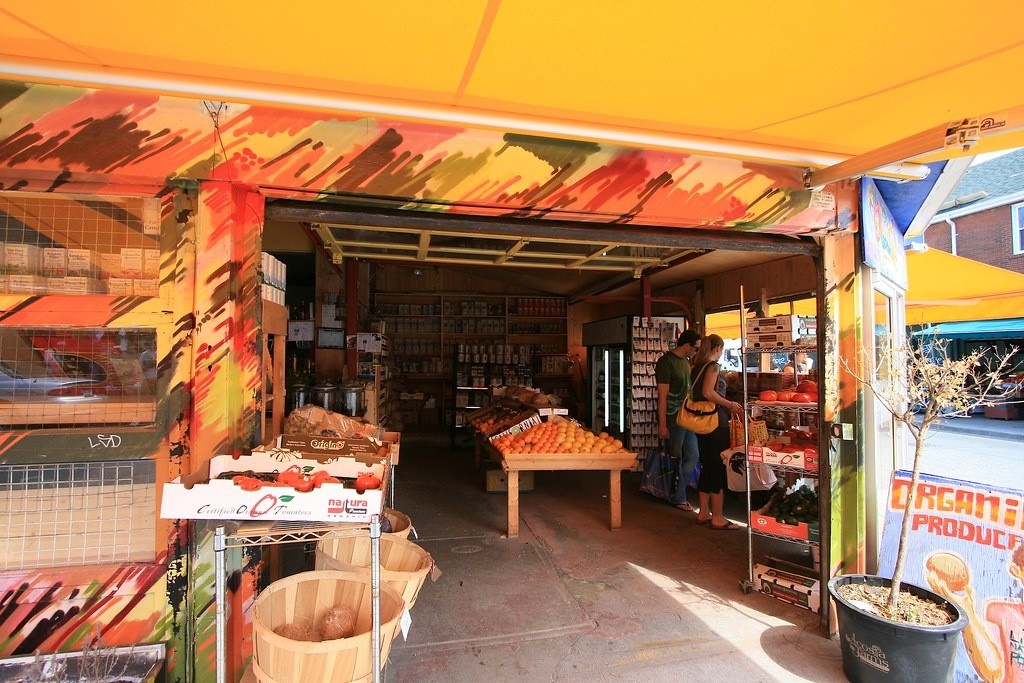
[399,394,438,425]
[750,511,821,544]
[382,303,506,356]
[737,372,804,392]
[485,458,535,492]
[470,397,541,445]
[746,315,817,349]
[159,431,401,525]
[749,446,820,470]
[0,243,160,294]
[752,555,820,613]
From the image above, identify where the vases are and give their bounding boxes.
[827,573,968,683]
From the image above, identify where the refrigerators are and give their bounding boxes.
[582,316,686,478]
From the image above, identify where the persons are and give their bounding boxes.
[655,330,700,511]
[783,350,813,374]
[689,334,743,528]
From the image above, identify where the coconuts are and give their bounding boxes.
[274,604,355,641]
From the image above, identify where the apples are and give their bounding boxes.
[232,470,379,492]
[473,417,509,433]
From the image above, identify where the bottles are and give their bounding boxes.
[292,307,299,321]
[299,299,306,320]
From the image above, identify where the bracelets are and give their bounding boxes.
[729,401,733,411]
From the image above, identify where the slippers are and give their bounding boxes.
[711,521,739,530]
[696,513,712,524]
[674,502,693,511]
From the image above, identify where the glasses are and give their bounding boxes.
[692,345,700,352]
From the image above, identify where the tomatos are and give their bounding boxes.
[764,484,818,526]
[766,429,819,454]
[759,380,818,403]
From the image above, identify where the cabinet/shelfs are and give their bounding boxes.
[740,285,823,616]
[0,183,195,683]
[374,291,569,427]
[260,299,288,450]
[356,332,392,429]
[450,344,533,452]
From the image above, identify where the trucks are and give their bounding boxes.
[718,347,781,373]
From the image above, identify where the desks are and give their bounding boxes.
[212,463,395,683]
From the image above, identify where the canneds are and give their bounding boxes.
[456,344,527,364]
[516,298,566,318]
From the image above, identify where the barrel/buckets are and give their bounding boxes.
[285,381,311,416]
[313,379,338,412]
[339,382,365,416]
[252,506,432,683]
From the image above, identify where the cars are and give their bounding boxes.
[0,359,100,398]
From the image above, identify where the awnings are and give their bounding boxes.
[911,319,1024,340]
[705,243,1024,339]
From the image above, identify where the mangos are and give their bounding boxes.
[492,420,629,455]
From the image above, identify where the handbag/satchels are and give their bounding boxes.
[676,361,719,435]
[640,438,678,503]
[719,446,776,491]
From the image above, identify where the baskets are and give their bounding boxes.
[728,410,768,445]
